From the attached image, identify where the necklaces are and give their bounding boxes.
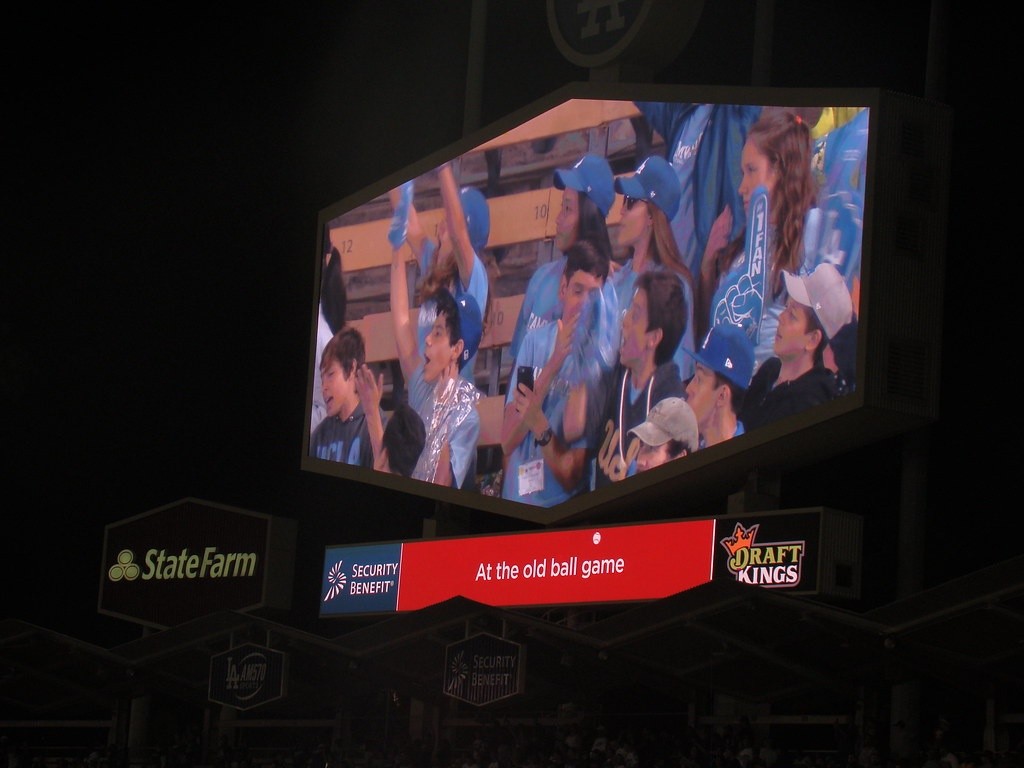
[620,369,655,468]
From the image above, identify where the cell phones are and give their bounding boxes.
[516,366,534,412]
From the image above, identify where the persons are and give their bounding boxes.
[0,714,1024,768]
[310,98,866,510]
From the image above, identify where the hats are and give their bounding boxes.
[443,286,482,373]
[456,181,490,259]
[552,153,616,217]
[615,155,679,222]
[682,323,754,391]
[626,397,699,453]
[783,263,853,340]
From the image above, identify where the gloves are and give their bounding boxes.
[388,180,412,250]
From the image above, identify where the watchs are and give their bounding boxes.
[535,426,552,447]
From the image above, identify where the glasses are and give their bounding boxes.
[622,193,649,211]
